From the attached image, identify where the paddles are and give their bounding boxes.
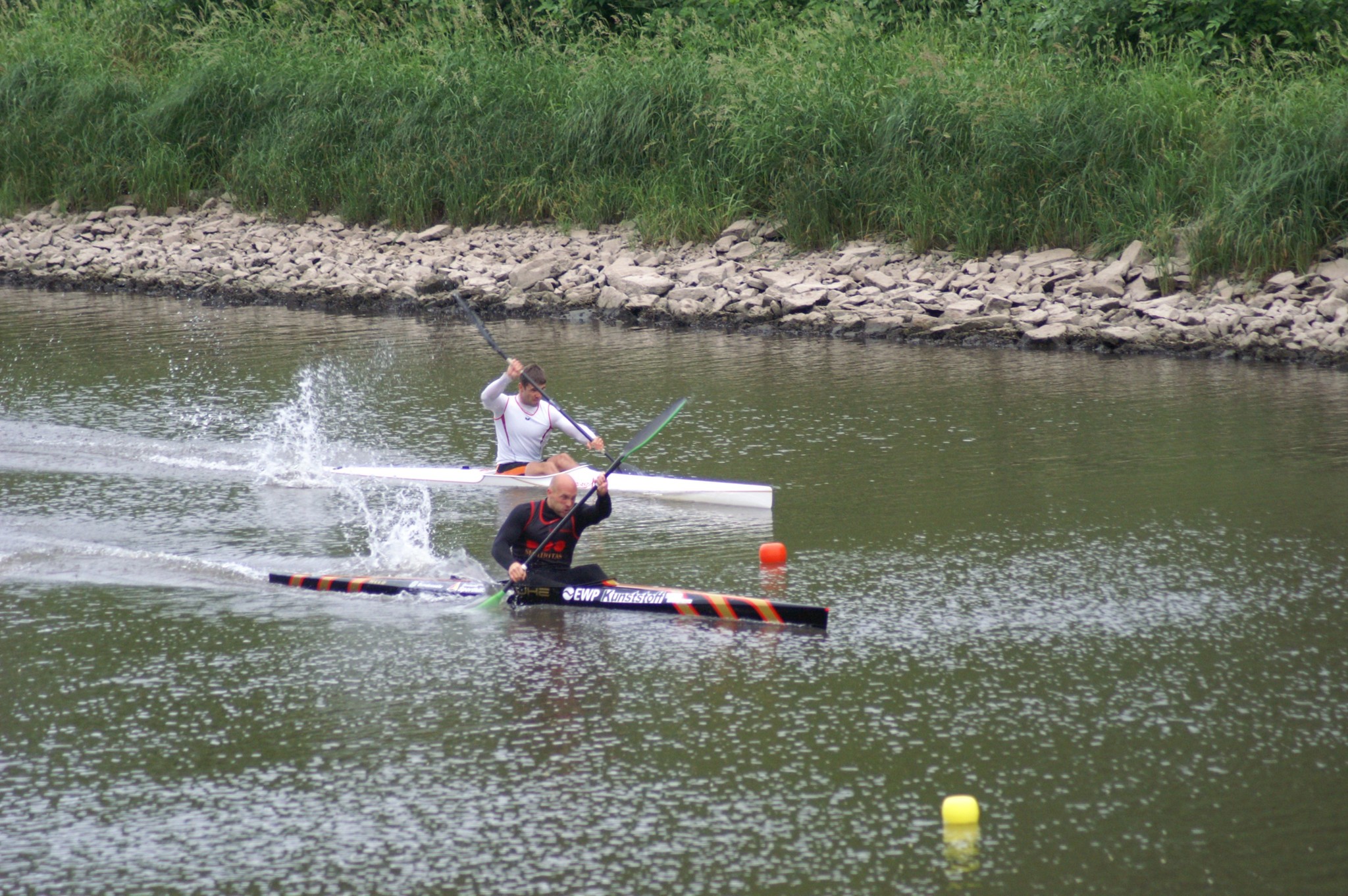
[478,395,687,607]
[470,308,644,476]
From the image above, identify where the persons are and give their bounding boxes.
[490,472,613,588]
[480,358,604,477]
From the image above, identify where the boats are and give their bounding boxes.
[269,572,829,628]
[325,464,772,510]
